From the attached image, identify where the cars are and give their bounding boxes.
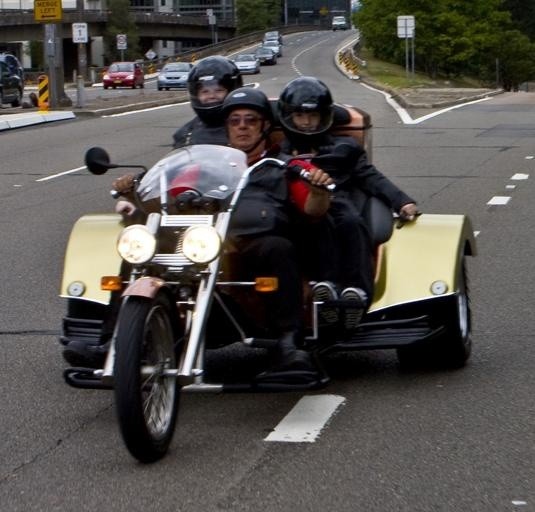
[102,61,144,89]
[332,16,348,31]
[232,31,283,75]
[0,53,25,107]
[156,62,193,91]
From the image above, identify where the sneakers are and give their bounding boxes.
[340,287,370,331]
[61,337,111,368]
[271,343,313,374]
[309,280,339,331]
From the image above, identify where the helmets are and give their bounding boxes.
[186,54,244,130]
[222,87,273,138]
[274,76,337,146]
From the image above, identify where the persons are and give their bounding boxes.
[109,86,336,378]
[172,55,244,151]
[275,74,423,331]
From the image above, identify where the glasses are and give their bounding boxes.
[224,115,264,127]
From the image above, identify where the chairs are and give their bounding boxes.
[172,101,392,248]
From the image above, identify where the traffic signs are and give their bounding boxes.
[34,1,62,22]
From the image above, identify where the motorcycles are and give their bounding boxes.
[57,97,477,462]
